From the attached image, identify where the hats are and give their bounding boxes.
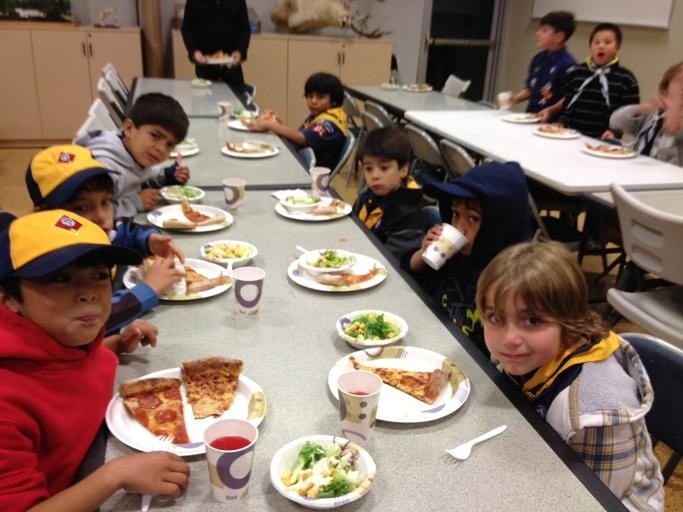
[419,161,530,208]
[0,207,146,278]
[24,143,123,207]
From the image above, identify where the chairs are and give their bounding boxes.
[328,119,683,512]
[73,63,130,147]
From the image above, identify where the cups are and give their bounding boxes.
[230,266,263,316]
[221,179,247,208]
[497,91,513,112]
[216,101,231,121]
[334,371,382,444]
[201,419,259,501]
[419,223,468,272]
[308,167,330,196]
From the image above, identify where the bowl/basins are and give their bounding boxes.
[279,196,318,214]
[270,433,376,510]
[298,248,356,273]
[160,186,205,204]
[336,309,407,349]
[199,240,257,267]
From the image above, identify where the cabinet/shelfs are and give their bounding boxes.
[171,25,393,152]
[0,19,147,148]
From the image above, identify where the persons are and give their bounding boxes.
[554,22,640,140]
[608,61,683,170]
[476,229,665,512]
[508,11,579,123]
[181,0,251,99]
[353,127,429,258]
[248,73,347,176]
[0,208,189,512]
[399,161,530,359]
[24,144,185,338]
[85,92,190,219]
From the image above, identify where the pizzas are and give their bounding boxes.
[119,376,189,446]
[183,265,228,295]
[227,143,262,155]
[585,142,629,155]
[317,266,378,286]
[349,356,451,406]
[180,201,226,228]
[314,199,336,215]
[181,355,242,420]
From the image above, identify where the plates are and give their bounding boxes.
[105,366,264,455]
[286,249,387,292]
[328,347,470,425]
[381,78,433,93]
[146,204,232,233]
[121,257,230,303]
[191,58,235,89]
[275,197,352,222]
[503,112,636,160]
[169,118,279,160]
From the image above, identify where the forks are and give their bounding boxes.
[140,435,174,511]
[444,425,509,462]
